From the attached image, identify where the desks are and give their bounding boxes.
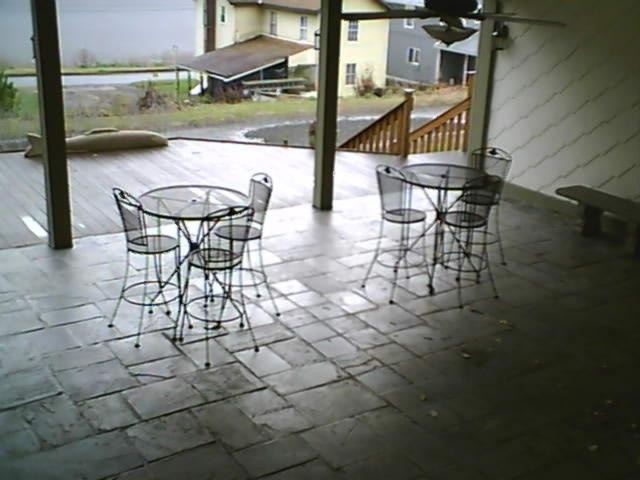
[139,184,253,328]
[399,161,490,286]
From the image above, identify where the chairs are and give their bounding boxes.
[360,164,435,305]
[172,205,260,364]
[448,144,509,266]
[204,170,282,317]
[107,185,194,347]
[427,172,501,302]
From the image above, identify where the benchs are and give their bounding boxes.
[555,185,639,253]
